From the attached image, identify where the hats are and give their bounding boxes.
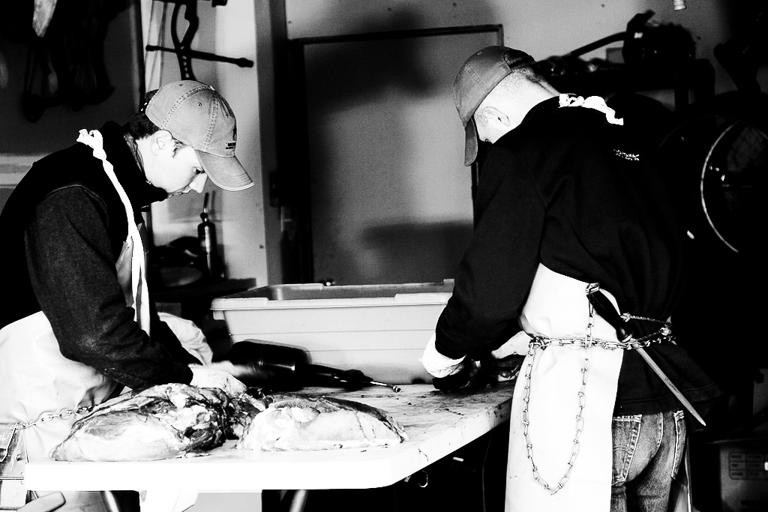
[144,79,254,191]
[455,46,533,165]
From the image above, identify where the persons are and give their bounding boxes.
[0,79,256,512]
[418,44,692,511]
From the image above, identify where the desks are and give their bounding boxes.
[25,383,515,512]
